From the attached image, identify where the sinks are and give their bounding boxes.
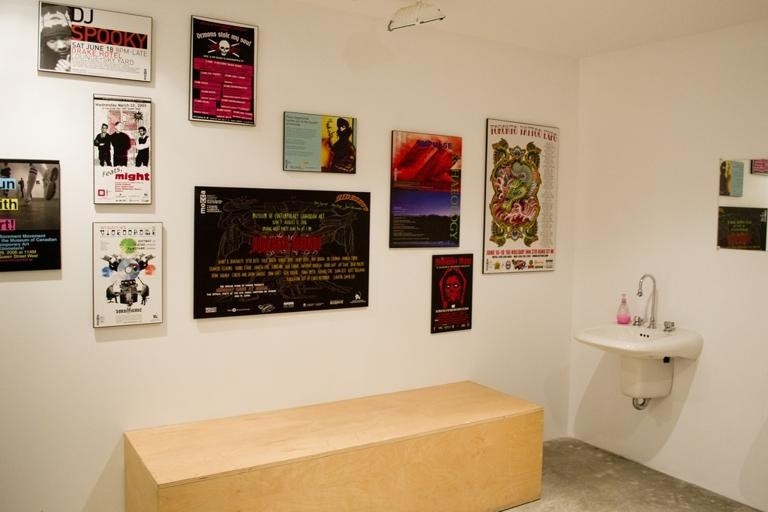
[576,322,699,362]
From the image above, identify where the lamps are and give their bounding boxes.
[387,0,447,32]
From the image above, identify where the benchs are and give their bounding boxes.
[125,378,544,512]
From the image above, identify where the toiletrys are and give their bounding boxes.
[617,292,631,324]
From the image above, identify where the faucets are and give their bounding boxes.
[635,274,656,330]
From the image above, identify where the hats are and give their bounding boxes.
[42,6,72,46]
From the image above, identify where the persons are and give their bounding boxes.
[110,122,131,167]
[0,162,11,198]
[18,177,26,198]
[40,6,72,71]
[94,123,111,165]
[131,126,149,166]
[24,164,40,202]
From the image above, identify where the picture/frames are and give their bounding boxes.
[189,15,259,126]
[36,0,152,83]
